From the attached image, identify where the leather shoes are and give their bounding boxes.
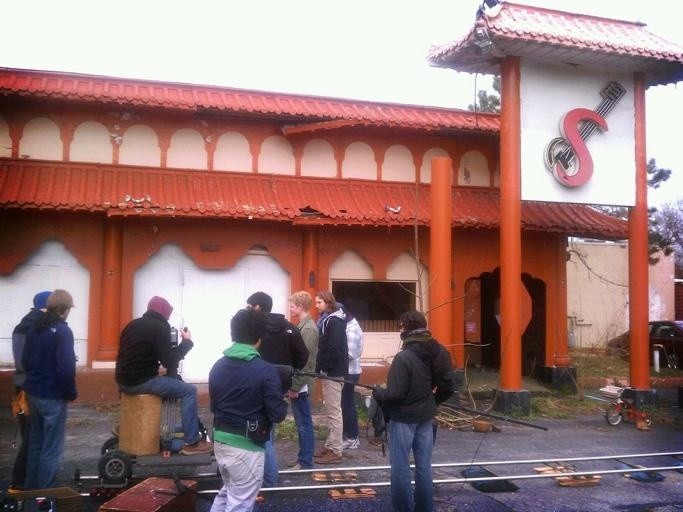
[314,446,329,457]
[314,448,342,463]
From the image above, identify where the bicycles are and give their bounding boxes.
[606,387,628,426]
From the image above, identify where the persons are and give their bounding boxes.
[246,290,362,489]
[209,309,288,512]
[12,290,78,512]
[373,311,453,512]
[114,296,214,456]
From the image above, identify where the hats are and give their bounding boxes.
[33,291,51,309]
[47,290,75,307]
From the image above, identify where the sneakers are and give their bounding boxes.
[287,463,312,471]
[286,459,298,467]
[110,422,118,437]
[342,437,357,449]
[180,439,213,454]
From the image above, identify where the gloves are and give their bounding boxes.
[372,383,383,401]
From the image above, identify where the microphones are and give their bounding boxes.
[273,364,294,377]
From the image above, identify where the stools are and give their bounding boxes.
[118,391,162,455]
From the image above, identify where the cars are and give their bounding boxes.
[607,319,683,370]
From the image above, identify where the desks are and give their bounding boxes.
[622,387,683,421]
